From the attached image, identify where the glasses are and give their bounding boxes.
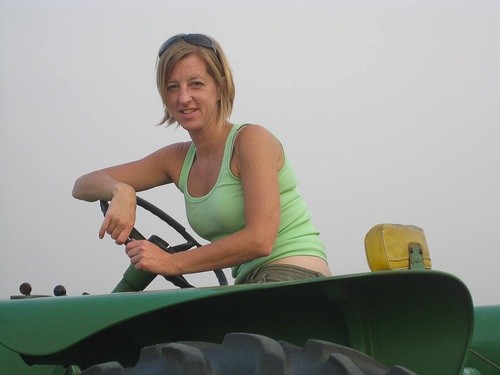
[157,34,220,60]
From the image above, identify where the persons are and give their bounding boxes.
[71,32,332,285]
